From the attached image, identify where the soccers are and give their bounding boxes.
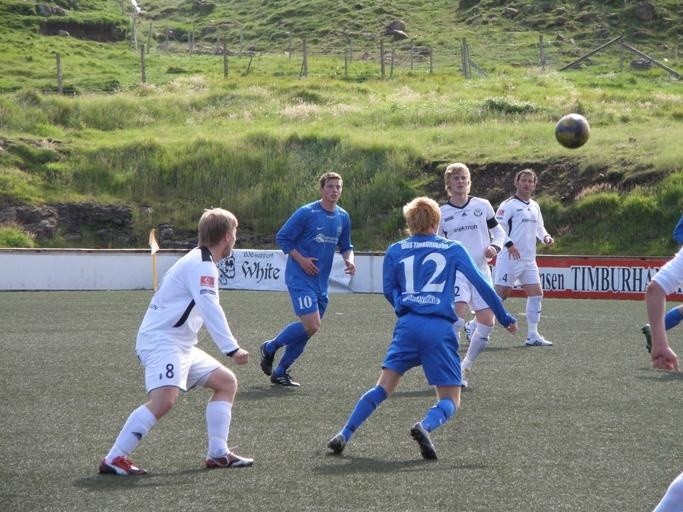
[555,112,590,149]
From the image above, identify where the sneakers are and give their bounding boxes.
[328,433,346,454]
[271,369,300,386]
[526,334,552,345]
[460,362,470,388]
[411,422,437,460]
[642,324,653,354]
[100,452,148,476]
[464,321,475,346]
[207,452,254,468]
[260,340,275,376]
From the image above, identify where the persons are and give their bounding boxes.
[327,197,519,461]
[259,172,355,390]
[436,164,507,388]
[640,211,683,352]
[644,242,683,375]
[99,208,253,477]
[465,169,554,347]
